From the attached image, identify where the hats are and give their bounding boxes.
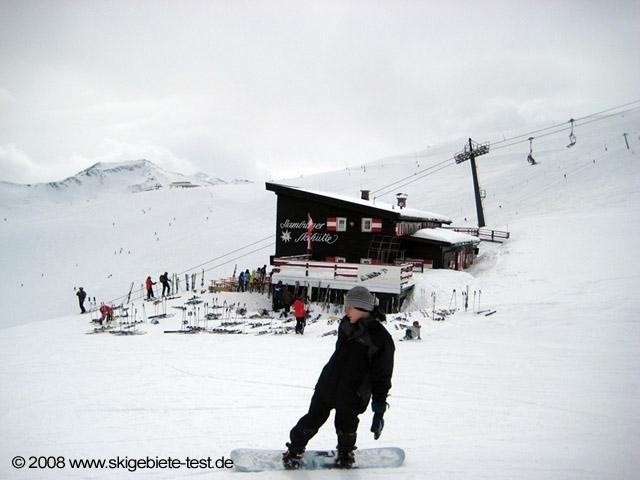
[345,287,375,311]
[413,321,418,325]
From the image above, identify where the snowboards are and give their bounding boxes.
[231,447,404,472]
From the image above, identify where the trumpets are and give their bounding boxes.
[323,316,342,336]
[420,307,457,321]
[391,311,414,331]
[86,264,322,335]
[476,310,496,316]
[323,284,343,314]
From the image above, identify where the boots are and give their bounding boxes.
[335,446,357,466]
[282,444,306,469]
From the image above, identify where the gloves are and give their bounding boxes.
[371,414,384,440]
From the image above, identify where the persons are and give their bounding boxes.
[76,287,87,314]
[282,284,395,470]
[271,279,297,317]
[98,304,114,325]
[292,293,306,335]
[399,321,422,341]
[237,264,268,293]
[162,271,171,297]
[145,276,157,299]
